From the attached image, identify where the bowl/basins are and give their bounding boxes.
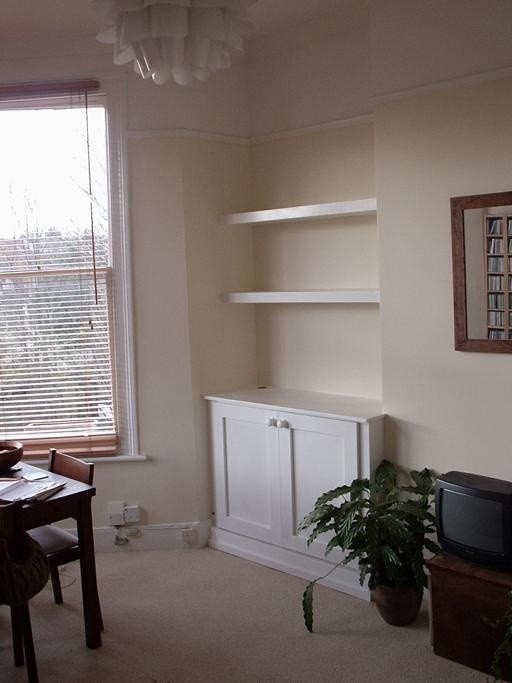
[0,439,23,471]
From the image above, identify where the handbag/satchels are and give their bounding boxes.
[1,533,49,604]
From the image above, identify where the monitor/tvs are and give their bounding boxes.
[435,469,512,575]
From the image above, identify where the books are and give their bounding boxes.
[0,476,68,501]
[485,216,512,339]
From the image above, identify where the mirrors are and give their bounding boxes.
[450,189,512,352]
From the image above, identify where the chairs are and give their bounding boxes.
[1,497,53,681]
[5,447,105,668]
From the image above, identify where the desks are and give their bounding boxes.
[1,461,103,650]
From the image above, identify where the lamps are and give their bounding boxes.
[95,0,244,87]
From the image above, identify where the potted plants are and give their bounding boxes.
[299,454,438,632]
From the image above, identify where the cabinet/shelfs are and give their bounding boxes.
[482,213,512,341]
[203,383,386,602]
[217,192,380,304]
[427,551,512,681]
